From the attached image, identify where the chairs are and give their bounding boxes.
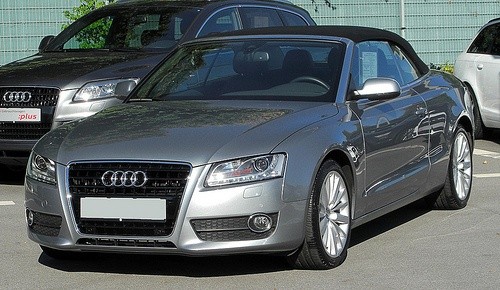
[229,51,270,91]
[319,47,356,95]
[354,45,388,87]
[141,30,168,47]
[282,49,314,83]
[176,16,216,42]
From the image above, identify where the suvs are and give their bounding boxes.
[0,0,317,173]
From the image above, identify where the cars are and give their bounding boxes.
[24,25,475,270]
[452,17,500,139]
